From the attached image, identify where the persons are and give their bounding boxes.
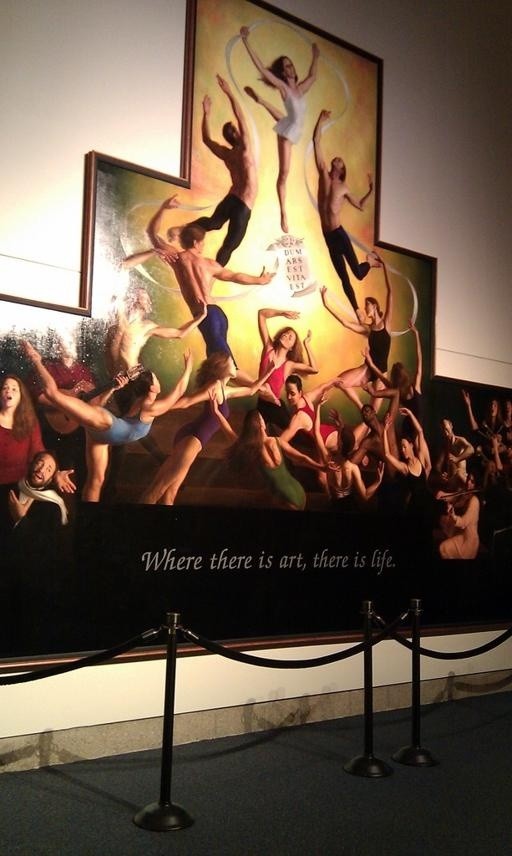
[239,25,321,233]
[167,71,260,268]
[312,108,382,326]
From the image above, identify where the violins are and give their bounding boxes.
[448,489,472,514]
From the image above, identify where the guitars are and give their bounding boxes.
[44,363,145,434]
